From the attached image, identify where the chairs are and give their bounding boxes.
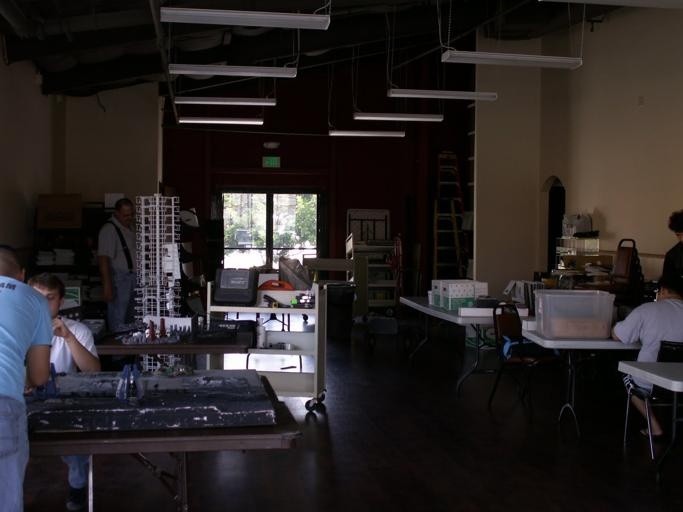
[257,280,297,333]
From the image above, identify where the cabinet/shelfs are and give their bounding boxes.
[32,209,119,323]
[466,103,476,280]
[136,193,180,372]
[205,280,327,412]
[346,232,402,321]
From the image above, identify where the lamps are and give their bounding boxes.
[159,0,333,34]
[176,81,265,126]
[384,6,500,102]
[325,63,407,138]
[169,23,301,79]
[435,0,586,70]
[350,46,446,123]
[171,78,277,108]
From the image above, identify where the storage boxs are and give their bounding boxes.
[214,269,260,305]
[37,192,83,229]
[79,319,106,343]
[48,273,82,312]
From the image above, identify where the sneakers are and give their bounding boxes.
[67,486,86,512]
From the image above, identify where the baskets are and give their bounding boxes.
[443,296,479,311]
[442,280,488,298]
[432,280,442,295]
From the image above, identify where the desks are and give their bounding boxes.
[87,320,258,371]
[26,374,302,512]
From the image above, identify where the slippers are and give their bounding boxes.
[638,428,662,441]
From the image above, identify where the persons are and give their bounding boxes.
[612,274,683,441]
[658,210,683,283]
[27,272,101,510]
[97,198,136,336]
[0,245,51,512]
